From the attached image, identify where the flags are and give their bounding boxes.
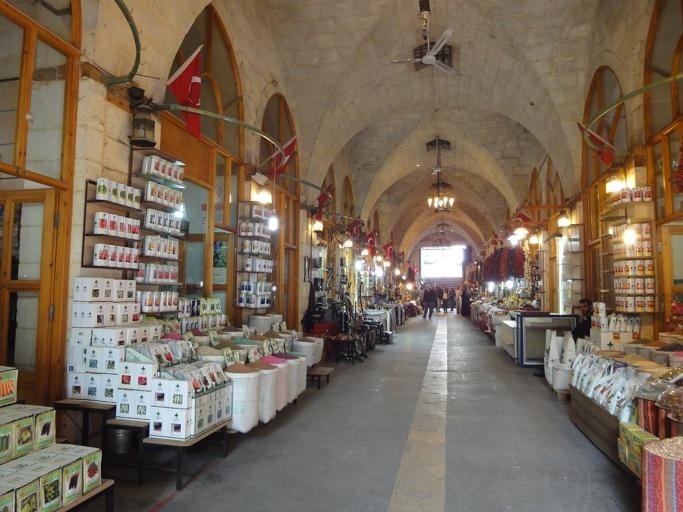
[314,181,334,221]
[265,138,297,180]
[512,210,531,229]
[491,232,504,246]
[165,45,204,141]
[347,217,419,278]
[575,122,614,167]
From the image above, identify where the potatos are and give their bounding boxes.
[0,378,14,397]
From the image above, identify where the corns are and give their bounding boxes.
[0,434,9,451]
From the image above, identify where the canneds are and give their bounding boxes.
[135,155,183,312]
[620,187,652,202]
[92,178,142,271]
[611,222,654,256]
[613,260,655,313]
[178,298,201,333]
[590,303,607,330]
[239,205,273,308]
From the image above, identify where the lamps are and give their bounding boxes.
[557,207,570,227]
[344,231,353,249]
[427,137,456,247]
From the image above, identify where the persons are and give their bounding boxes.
[531,297,592,378]
[411,280,471,320]
[495,297,509,311]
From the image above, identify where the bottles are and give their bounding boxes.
[238,204,274,309]
[608,187,657,314]
[177,293,229,335]
[93,154,186,315]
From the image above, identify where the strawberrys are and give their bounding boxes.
[89,462,98,478]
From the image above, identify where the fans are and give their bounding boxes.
[394,16,459,74]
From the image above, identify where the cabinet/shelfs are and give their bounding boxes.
[599,200,664,315]
[307,367,334,389]
[54,398,232,512]
[236,202,273,309]
[130,147,188,314]
[81,179,141,272]
